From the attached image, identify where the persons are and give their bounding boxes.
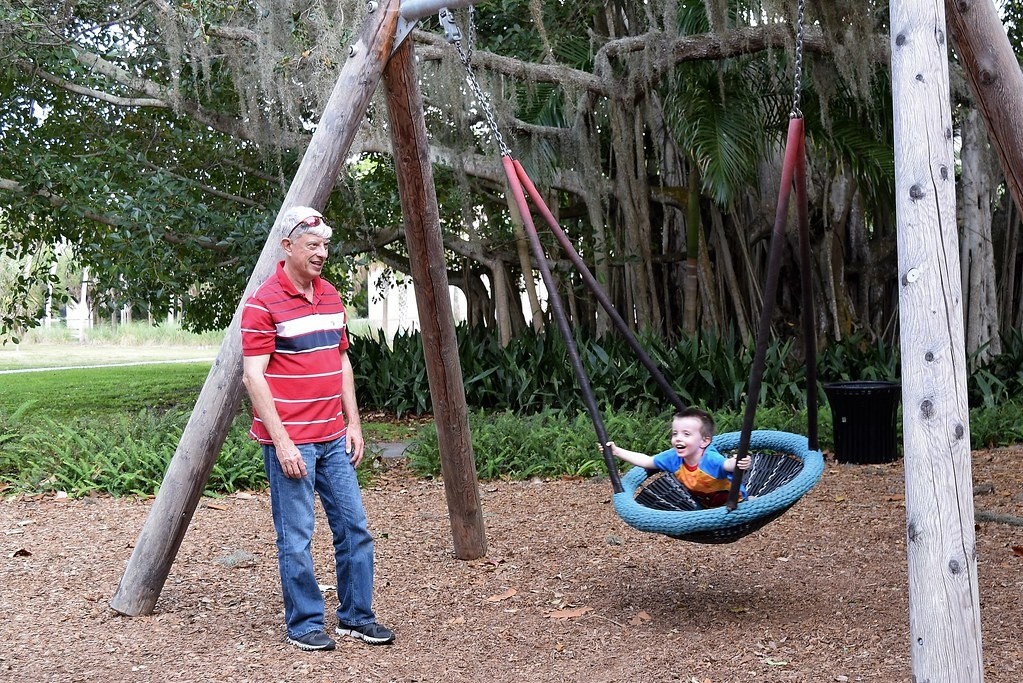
[238,206,396,650]
[597,408,752,511]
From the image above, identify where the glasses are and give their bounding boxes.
[287,216,330,237]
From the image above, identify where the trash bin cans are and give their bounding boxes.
[821,382,901,466]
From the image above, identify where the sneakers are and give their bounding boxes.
[286,630,336,651]
[335,619,396,645]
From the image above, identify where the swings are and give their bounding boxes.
[434,0,825,546]
